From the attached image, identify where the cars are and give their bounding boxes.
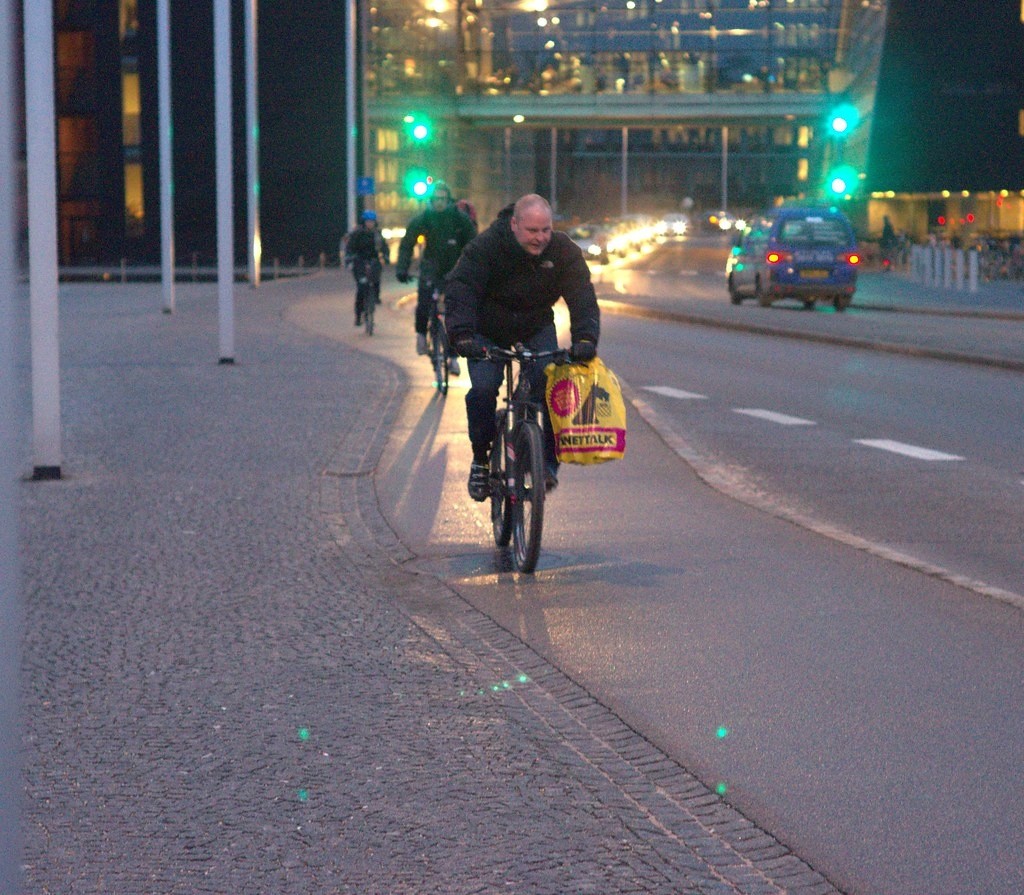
[567,210,692,266]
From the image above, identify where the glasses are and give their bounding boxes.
[431,196,445,200]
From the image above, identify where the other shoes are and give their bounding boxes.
[418,335,426,354]
[450,359,459,375]
[355,317,362,326]
[375,299,381,305]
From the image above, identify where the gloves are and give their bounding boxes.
[569,342,597,361]
[396,271,411,282]
[457,336,487,357]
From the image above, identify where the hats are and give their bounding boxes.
[362,211,376,219]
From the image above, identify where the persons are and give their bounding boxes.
[444,194,600,503]
[344,211,389,326]
[882,215,1024,279]
[396,184,477,376]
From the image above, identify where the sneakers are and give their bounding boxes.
[468,462,490,502]
[544,466,558,490]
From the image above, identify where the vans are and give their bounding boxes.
[723,205,863,313]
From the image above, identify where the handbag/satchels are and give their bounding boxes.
[544,357,627,464]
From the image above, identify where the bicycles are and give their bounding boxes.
[408,274,458,394]
[449,339,590,572]
[353,258,379,336]
